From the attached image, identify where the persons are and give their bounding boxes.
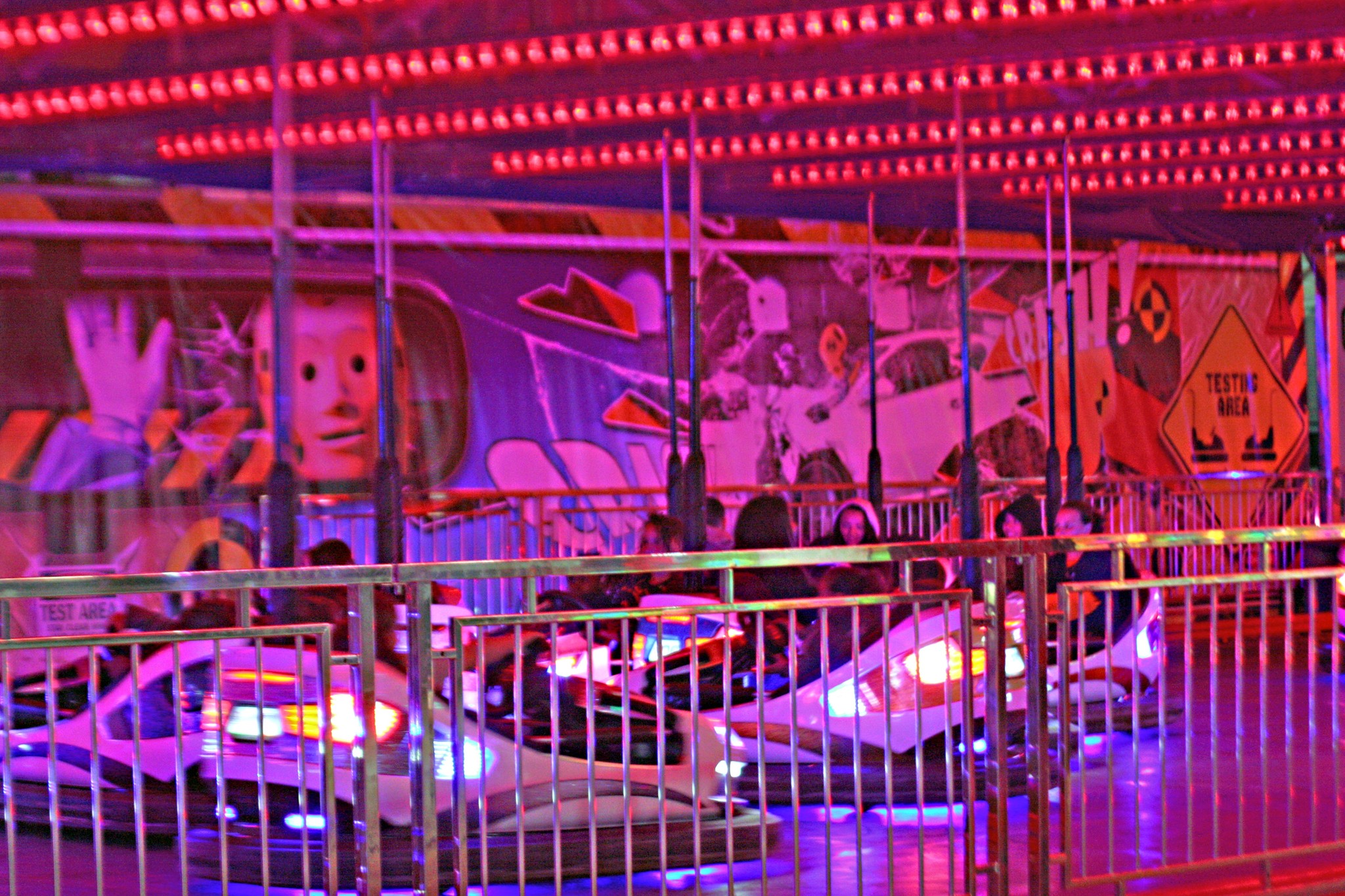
[295,537,396,658]
[569,549,608,594]
[639,512,684,594]
[706,495,736,550]
[765,566,888,678]
[806,498,893,591]
[1047,500,1150,665]
[177,600,236,629]
[994,494,1048,593]
